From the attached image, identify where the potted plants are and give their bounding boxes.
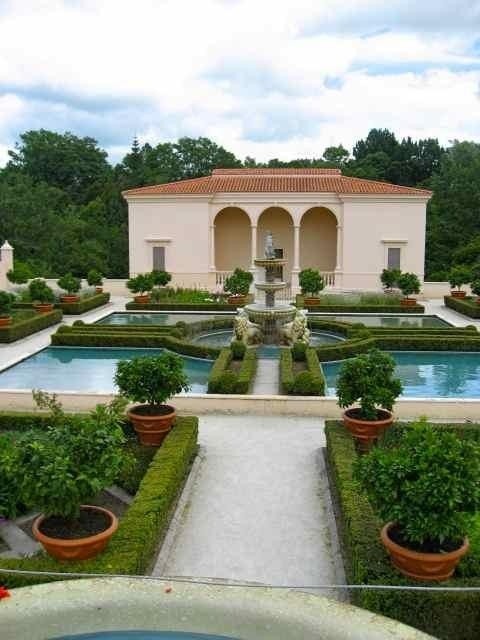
[334,348,479,582]
[380,265,480,309]
[3,354,193,561]
[222,267,326,305]
[0,269,171,327]
